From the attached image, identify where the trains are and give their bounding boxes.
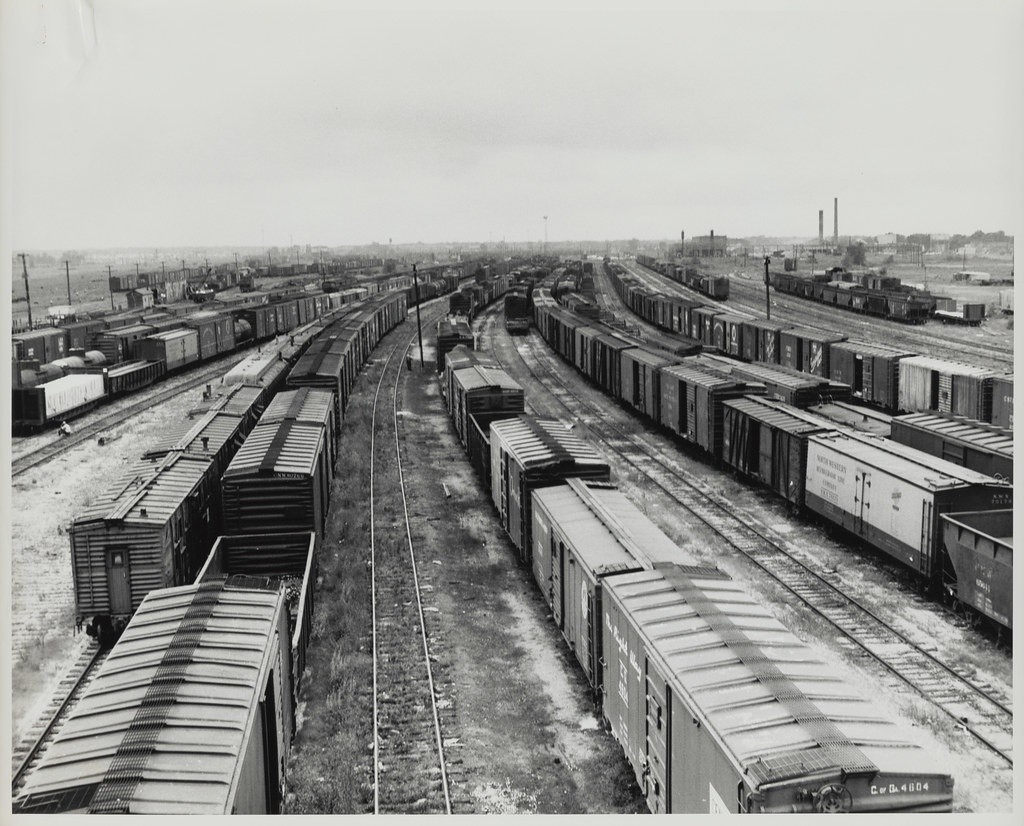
[11,249,1015,814]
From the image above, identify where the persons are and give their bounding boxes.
[58,421,70,436]
[406,353,412,371]
